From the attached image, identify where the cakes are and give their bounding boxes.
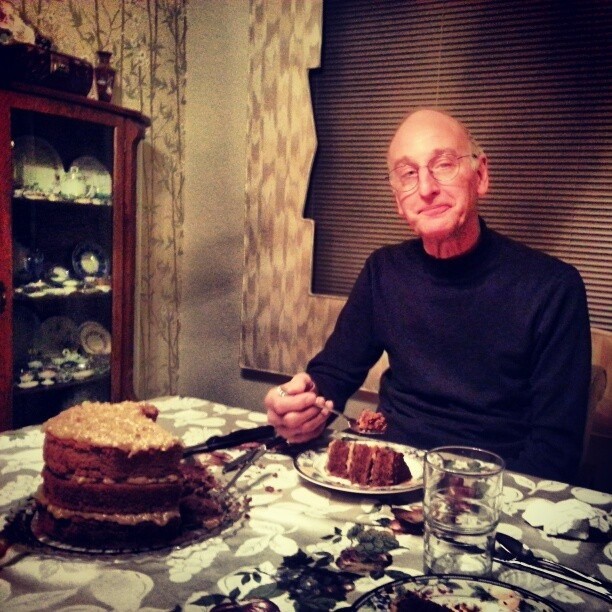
[323,438,407,486]
[32,399,227,548]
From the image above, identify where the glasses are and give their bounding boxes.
[384,154,478,193]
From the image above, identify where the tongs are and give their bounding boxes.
[184,424,286,501]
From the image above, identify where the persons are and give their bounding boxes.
[265,109,591,483]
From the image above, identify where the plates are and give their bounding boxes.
[72,240,111,279]
[60,155,113,197]
[40,317,81,354]
[293,440,444,496]
[14,134,66,194]
[350,574,558,612]
[80,324,112,356]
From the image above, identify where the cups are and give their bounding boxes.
[423,445,508,579]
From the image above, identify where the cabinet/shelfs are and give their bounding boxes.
[1,83,152,413]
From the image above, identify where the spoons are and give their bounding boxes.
[493,530,601,584]
[318,404,388,436]
